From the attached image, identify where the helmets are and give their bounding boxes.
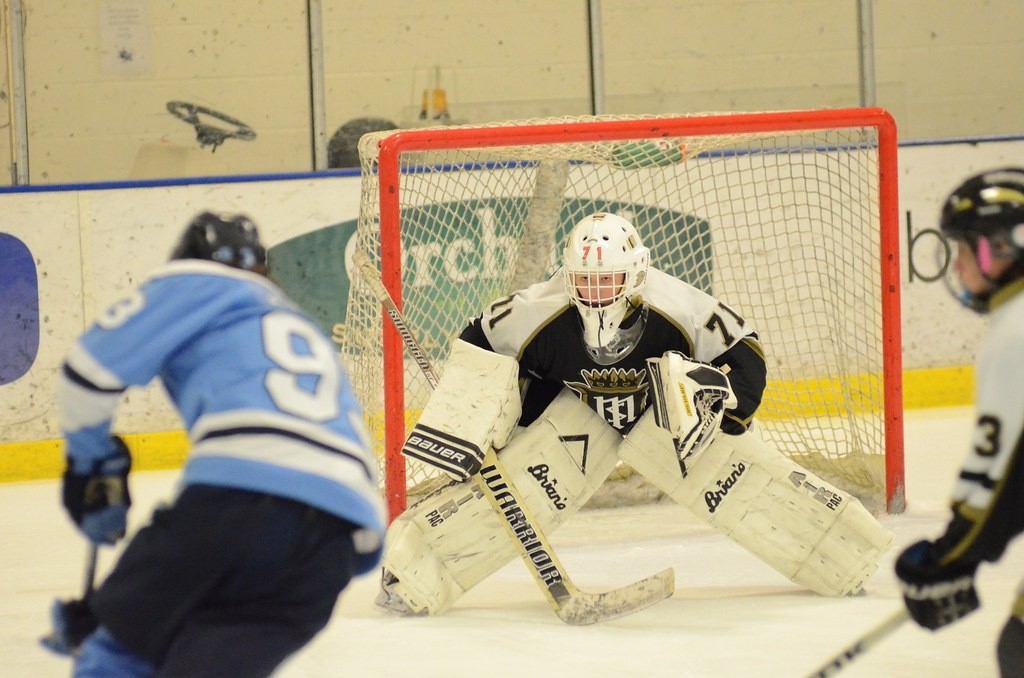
[563,212,650,349]
[171,209,268,276]
[935,165,1024,317]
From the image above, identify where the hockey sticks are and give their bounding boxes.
[80,482,108,602]
[349,250,690,627]
[808,607,912,677]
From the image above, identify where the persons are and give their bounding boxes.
[893,167,1024,677]
[376,210,893,626]
[42,211,391,678]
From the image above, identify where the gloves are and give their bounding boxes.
[61,435,133,543]
[896,542,982,631]
[61,594,102,646]
